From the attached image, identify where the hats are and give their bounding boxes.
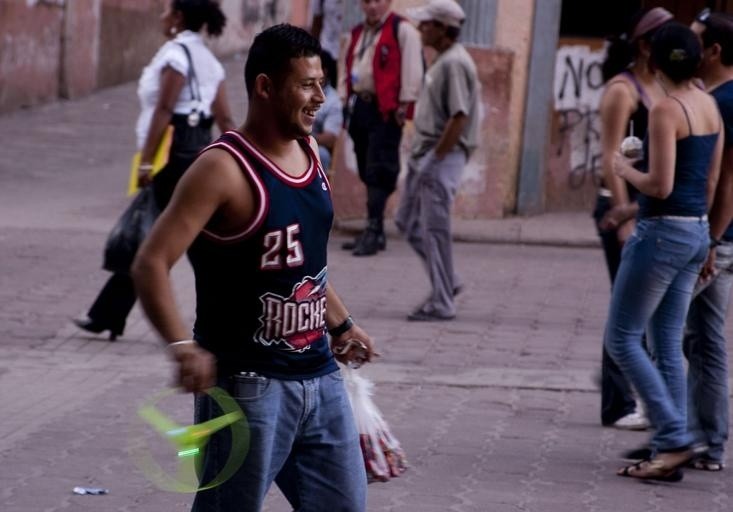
[408,1,464,28]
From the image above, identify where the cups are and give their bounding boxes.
[621,135,644,162]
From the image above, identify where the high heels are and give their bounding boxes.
[71,315,121,340]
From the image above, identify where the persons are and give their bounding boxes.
[681,11,733,471]
[72,0,238,341]
[603,22,724,483]
[590,4,679,429]
[335,1,427,257]
[128,24,377,512]
[395,1,481,324]
[307,47,343,174]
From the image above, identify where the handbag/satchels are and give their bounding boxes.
[155,107,217,180]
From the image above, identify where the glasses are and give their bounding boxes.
[699,7,712,25]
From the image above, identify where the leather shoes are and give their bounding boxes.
[342,228,386,256]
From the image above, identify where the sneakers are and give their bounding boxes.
[407,287,460,321]
[613,400,724,481]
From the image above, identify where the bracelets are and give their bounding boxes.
[398,108,408,119]
[327,315,355,338]
[165,339,197,350]
[708,235,722,249]
[136,164,154,171]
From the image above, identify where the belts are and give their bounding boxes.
[598,188,613,197]
[649,215,708,221]
[356,91,376,105]
